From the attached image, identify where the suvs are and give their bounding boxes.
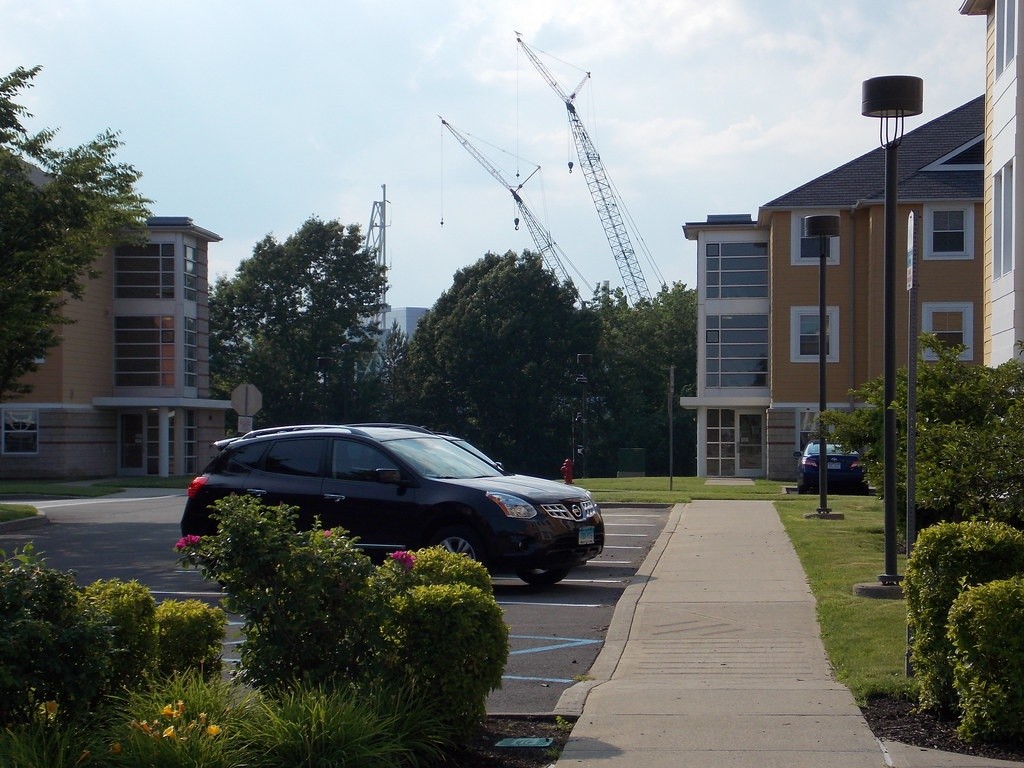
[181,423,606,587]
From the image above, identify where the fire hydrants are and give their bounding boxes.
[560,458,575,485]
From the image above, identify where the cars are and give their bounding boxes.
[793,439,870,496]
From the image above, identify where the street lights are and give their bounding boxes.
[853,75,925,599]
[803,214,846,520]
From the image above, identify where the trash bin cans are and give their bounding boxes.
[351,540,405,565]
